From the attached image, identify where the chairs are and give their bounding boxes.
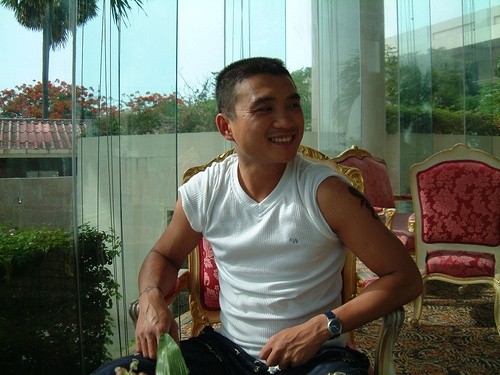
[409,143,500,333]
[333,146,416,256]
[130,145,404,375]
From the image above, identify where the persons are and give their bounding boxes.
[90,55,423,374]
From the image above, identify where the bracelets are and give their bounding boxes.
[138,286,165,298]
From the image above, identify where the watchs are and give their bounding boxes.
[324,311,342,340]
